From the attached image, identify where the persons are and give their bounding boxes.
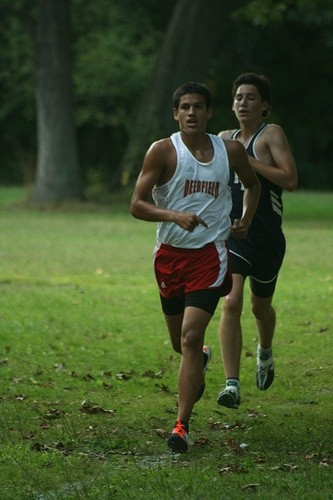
[217,72,299,410]
[129,80,262,454]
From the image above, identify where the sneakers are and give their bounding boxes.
[256,348,274,390]
[217,382,240,409]
[194,345,212,402]
[167,419,188,453]
